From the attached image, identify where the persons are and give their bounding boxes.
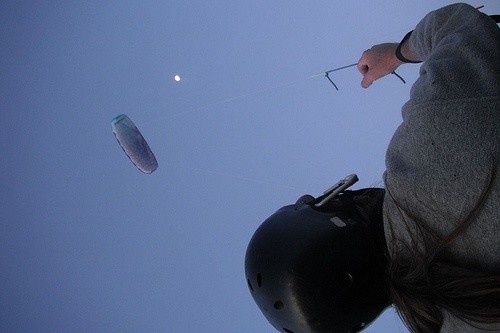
[243,4,500,333]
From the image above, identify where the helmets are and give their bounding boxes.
[241,174,400,332]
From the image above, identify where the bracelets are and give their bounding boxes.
[396,29,423,64]
